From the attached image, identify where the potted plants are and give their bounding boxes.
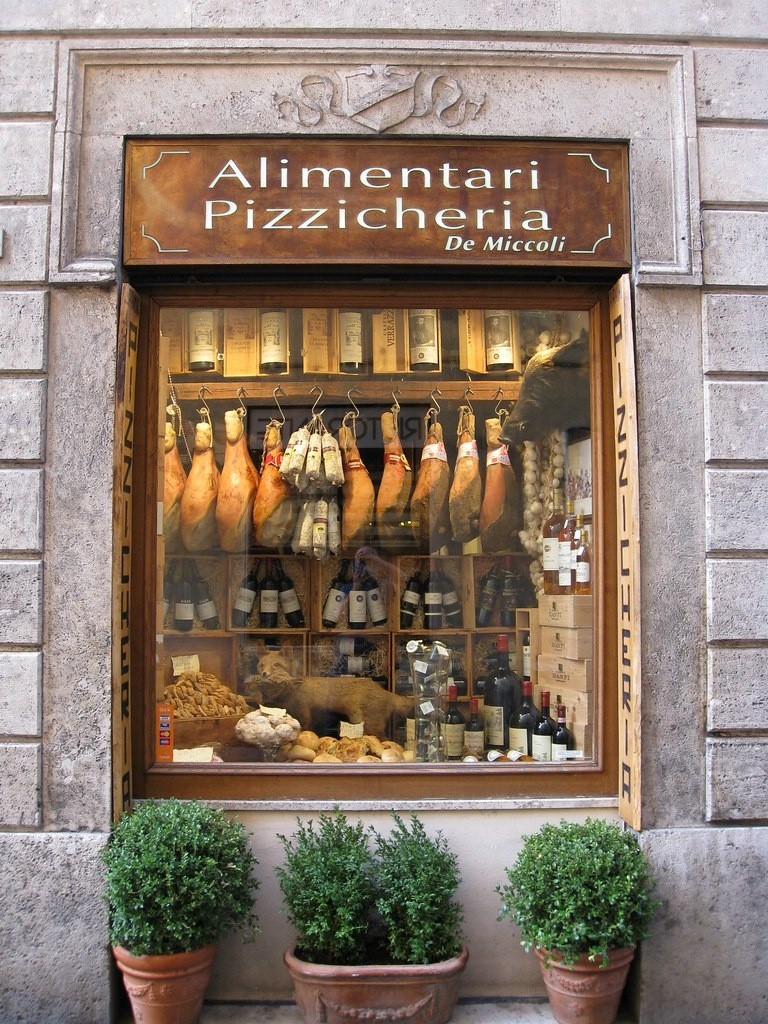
[276,808,470,1024]
[93,799,257,1024]
[493,814,661,1024]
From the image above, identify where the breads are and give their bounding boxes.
[273,732,416,763]
[234,704,301,744]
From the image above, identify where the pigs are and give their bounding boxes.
[498,336,589,447]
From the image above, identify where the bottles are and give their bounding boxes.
[186,306,217,371]
[408,306,439,372]
[259,307,288,372]
[464,696,485,762]
[461,753,478,763]
[163,560,538,698]
[543,487,592,595]
[509,681,541,758]
[338,308,366,375]
[484,634,522,751]
[551,705,575,761]
[531,690,558,762]
[440,685,465,760]
[505,749,537,762]
[485,748,513,762]
[483,308,513,370]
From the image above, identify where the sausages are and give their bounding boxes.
[279,427,346,560]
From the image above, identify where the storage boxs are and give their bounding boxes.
[153,436,594,763]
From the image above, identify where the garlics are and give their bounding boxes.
[518,432,567,596]
[521,324,571,374]
